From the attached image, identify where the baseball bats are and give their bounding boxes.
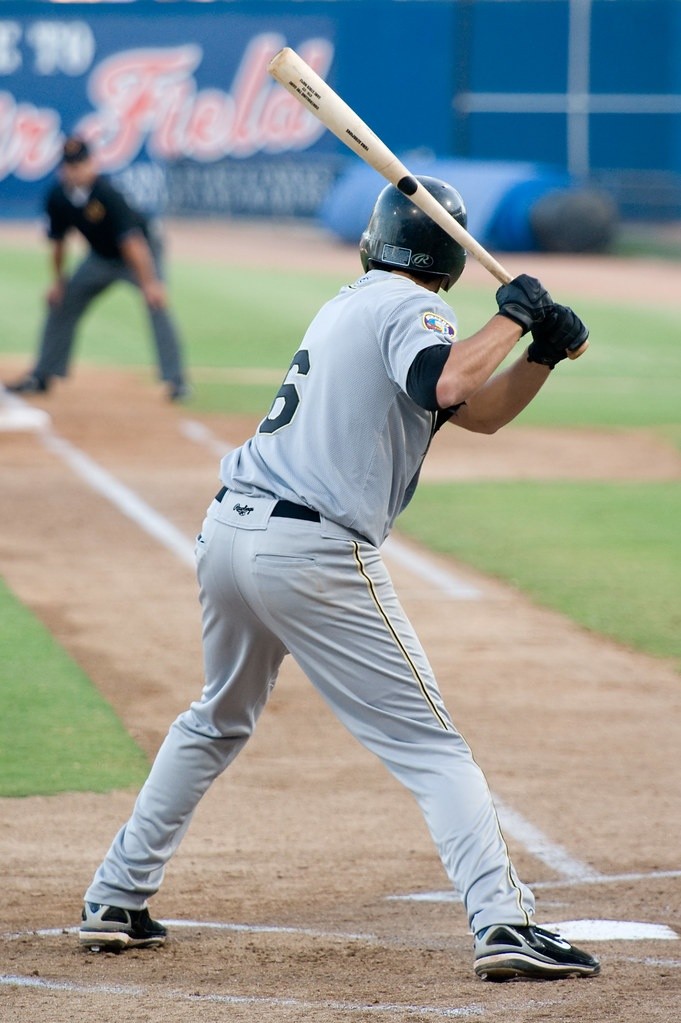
[267,46,589,360]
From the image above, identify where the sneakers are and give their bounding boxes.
[472,919,602,981]
[78,900,167,952]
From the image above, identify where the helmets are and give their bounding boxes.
[359,175,467,291]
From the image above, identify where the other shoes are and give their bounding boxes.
[5,378,49,394]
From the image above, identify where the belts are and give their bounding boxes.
[216,485,320,522]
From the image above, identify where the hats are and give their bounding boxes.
[63,140,88,163]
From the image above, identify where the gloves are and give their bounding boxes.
[527,303,588,371]
[495,273,555,338]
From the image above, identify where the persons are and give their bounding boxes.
[5,140,188,399]
[81,176,602,982]
